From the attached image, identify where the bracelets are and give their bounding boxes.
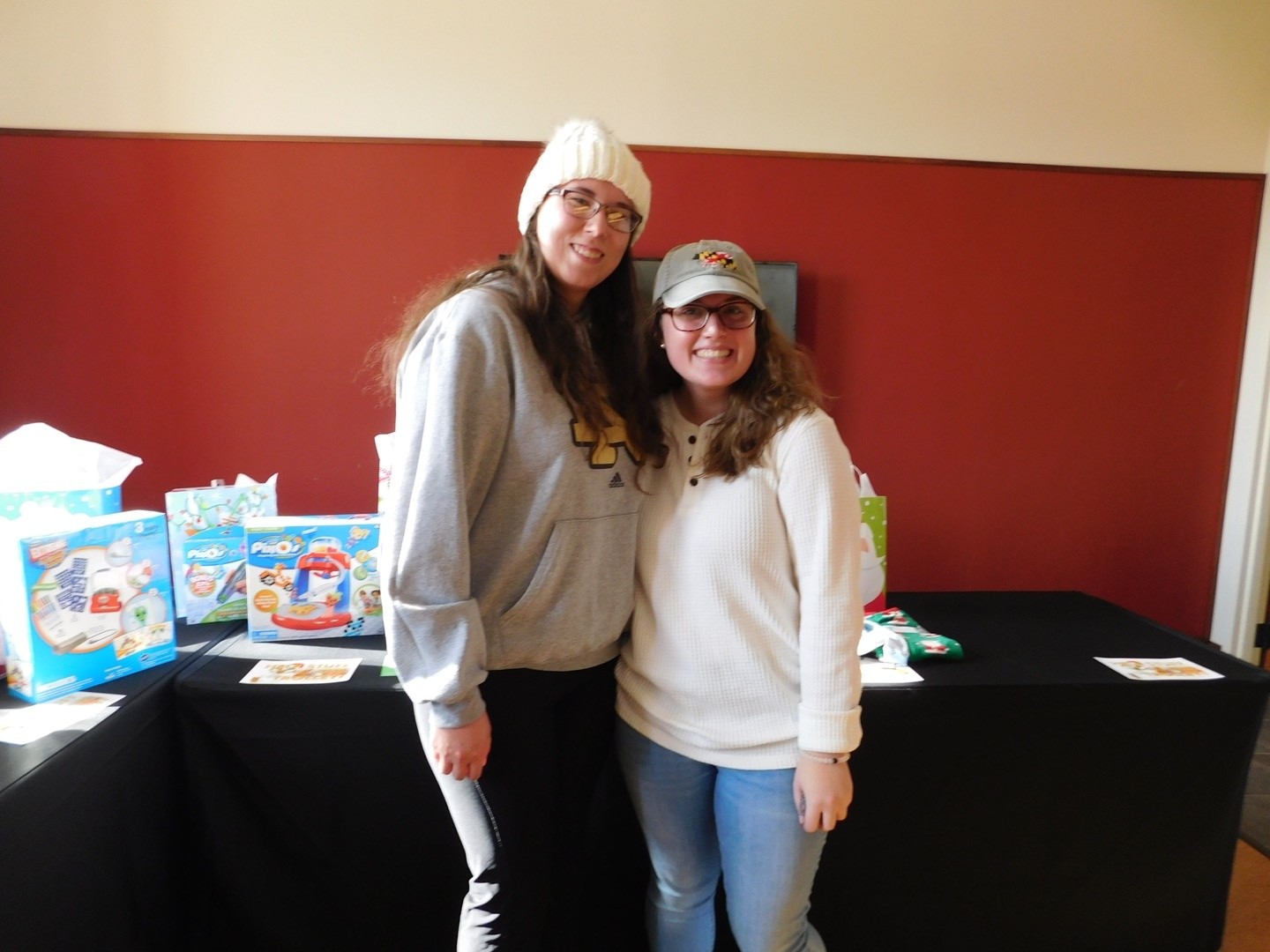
[800,749,851,765]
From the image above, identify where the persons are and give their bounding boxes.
[605,239,870,952]
[373,114,654,952]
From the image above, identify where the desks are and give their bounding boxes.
[0,620,248,952]
[170,586,1270,952]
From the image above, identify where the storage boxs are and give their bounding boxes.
[0,481,384,705]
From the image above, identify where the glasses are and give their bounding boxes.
[657,300,764,331]
[542,185,644,237]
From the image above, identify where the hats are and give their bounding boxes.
[514,121,651,249]
[648,236,769,313]
